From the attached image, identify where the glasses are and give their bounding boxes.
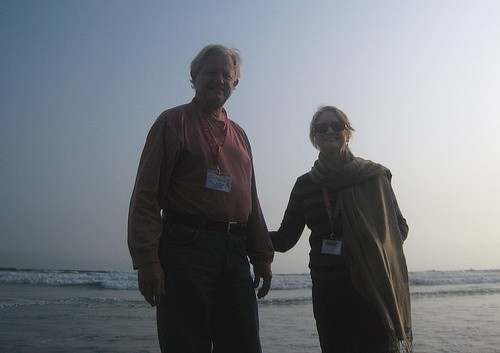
[312,121,349,133]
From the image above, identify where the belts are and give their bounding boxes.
[173,215,247,233]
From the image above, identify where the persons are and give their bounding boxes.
[269,105,414,353]
[126,44,273,353]
